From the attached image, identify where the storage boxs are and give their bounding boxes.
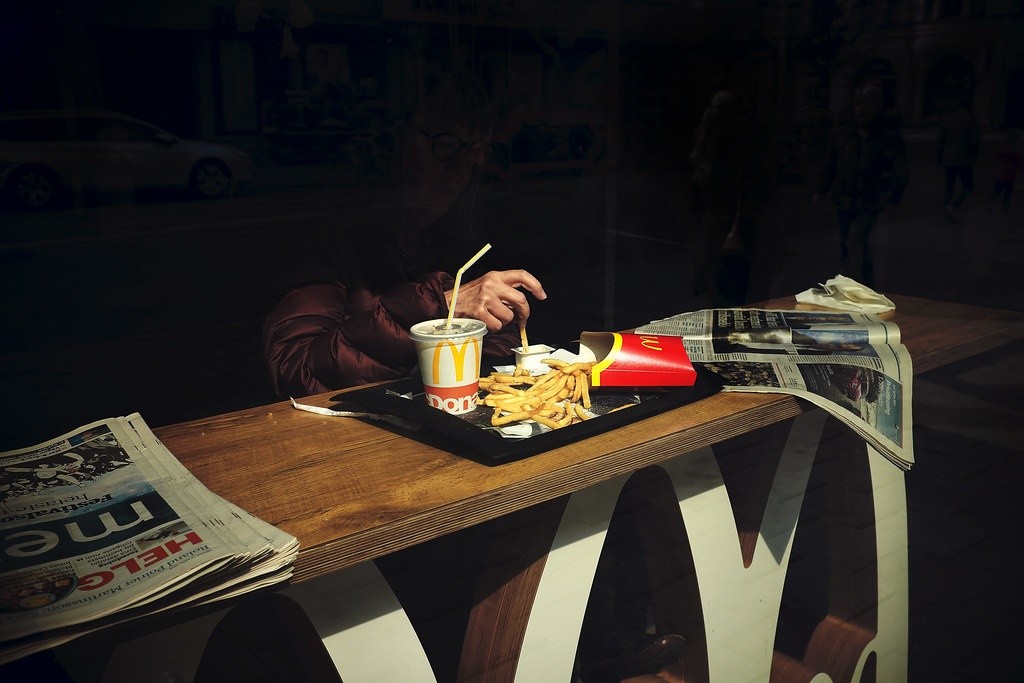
[579,331,697,393]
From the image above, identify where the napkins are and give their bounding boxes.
[795,273,896,314]
[483,400,600,438]
[492,348,578,376]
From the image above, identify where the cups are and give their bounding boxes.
[409,318,488,416]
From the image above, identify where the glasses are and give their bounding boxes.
[416,128,494,169]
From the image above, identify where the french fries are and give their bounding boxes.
[474,321,637,431]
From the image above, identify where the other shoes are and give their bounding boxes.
[579,629,687,683]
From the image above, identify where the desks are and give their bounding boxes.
[0,290,1024,683]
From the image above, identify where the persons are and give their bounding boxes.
[259,46,686,683]
[694,91,775,307]
[936,94,980,219]
[990,130,1024,211]
[812,59,909,289]
